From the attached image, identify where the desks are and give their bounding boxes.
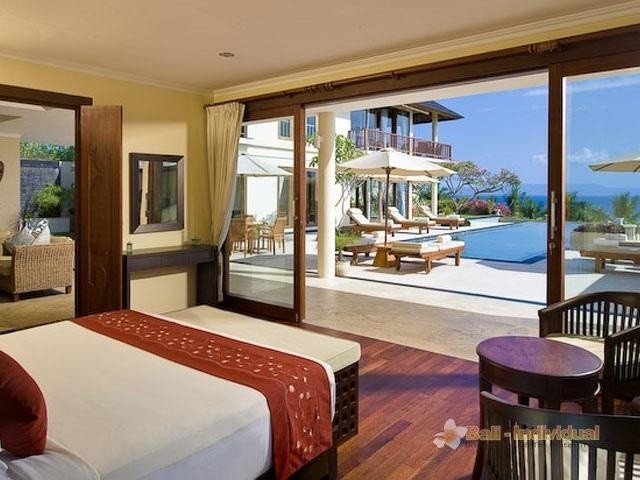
[472,335,604,422]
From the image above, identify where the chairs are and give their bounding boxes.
[331,203,468,274]
[360,136,444,160]
[223,212,289,260]
[471,388,639,480]
[536,286,640,417]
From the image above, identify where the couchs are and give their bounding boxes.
[0,230,76,303]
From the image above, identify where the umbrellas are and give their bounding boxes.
[338,147,457,247]
[236,152,295,215]
[359,174,441,209]
[588,148,640,176]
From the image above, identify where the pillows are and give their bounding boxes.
[1,218,52,255]
[1,347,47,458]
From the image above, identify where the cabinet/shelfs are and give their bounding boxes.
[120,242,219,312]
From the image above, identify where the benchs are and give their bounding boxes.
[578,233,640,270]
[161,303,363,448]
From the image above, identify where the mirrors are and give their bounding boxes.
[128,150,187,234]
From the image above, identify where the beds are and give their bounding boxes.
[1,307,340,480]
